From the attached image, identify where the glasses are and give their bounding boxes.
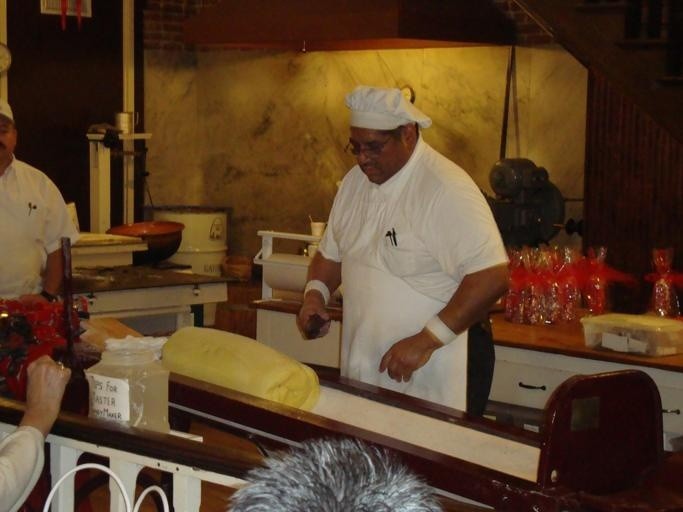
[343,136,392,158]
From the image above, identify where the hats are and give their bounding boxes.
[0,101,13,121]
[344,84,432,131]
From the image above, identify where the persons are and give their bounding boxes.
[296,84,514,416]
[0,95,83,307]
[0,354,71,512]
[225,435,448,512]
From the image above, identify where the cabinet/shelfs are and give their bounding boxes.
[487,344,683,435]
[254,308,339,367]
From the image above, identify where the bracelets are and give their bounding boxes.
[304,280,331,306]
[40,290,58,304]
[425,314,458,346]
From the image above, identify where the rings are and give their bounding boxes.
[56,360,65,370]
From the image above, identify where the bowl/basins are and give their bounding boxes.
[105,221,185,258]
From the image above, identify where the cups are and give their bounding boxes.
[310,223,326,239]
